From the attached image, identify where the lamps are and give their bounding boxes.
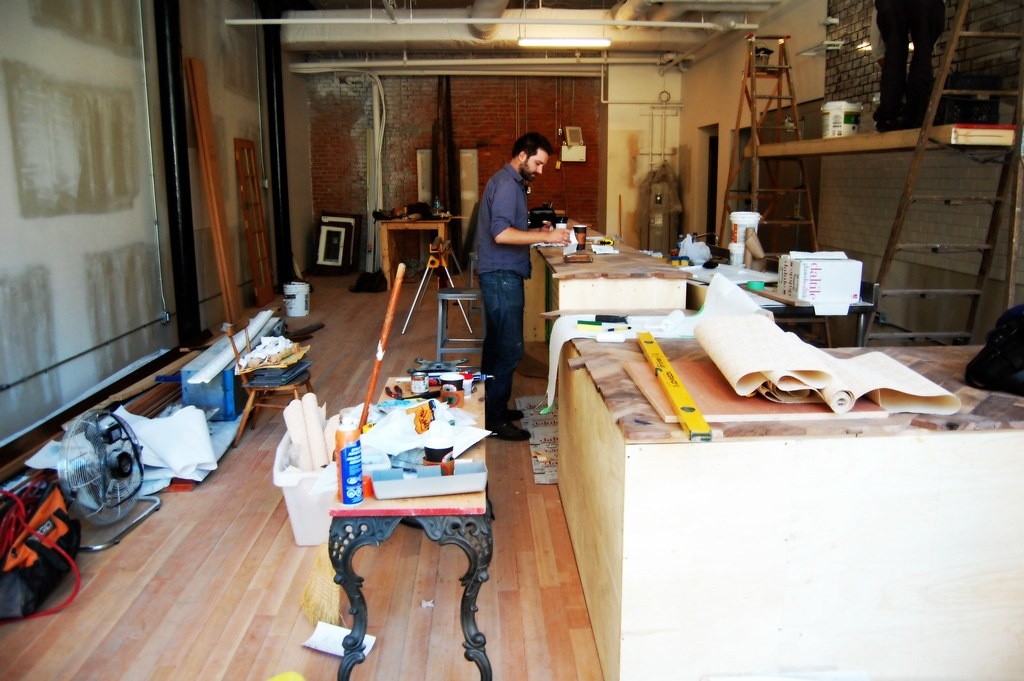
[517,0,613,48]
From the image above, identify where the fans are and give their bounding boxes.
[59,408,164,554]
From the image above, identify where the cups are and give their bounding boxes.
[439,374,464,391]
[556,223,566,229]
[573,225,587,251]
[440,388,464,408]
[422,457,455,475]
[423,442,454,463]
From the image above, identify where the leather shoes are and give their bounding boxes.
[486,422,530,441]
[508,410,523,421]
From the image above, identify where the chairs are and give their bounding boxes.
[222,318,313,448]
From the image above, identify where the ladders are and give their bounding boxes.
[860,1,1024,347]
[717,33,831,348]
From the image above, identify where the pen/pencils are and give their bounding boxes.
[608,327,631,331]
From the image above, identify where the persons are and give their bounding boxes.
[468,133,572,442]
[871,0,945,134]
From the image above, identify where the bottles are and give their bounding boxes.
[433,196,441,214]
[334,417,364,504]
[676,234,684,249]
[692,232,699,244]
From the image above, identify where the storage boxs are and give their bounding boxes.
[777,255,794,296]
[372,459,488,500]
[792,255,864,303]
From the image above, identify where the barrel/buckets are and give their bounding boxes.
[730,211,762,244]
[728,243,746,265]
[822,101,862,140]
[283,282,310,317]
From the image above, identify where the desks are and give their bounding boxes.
[535,219,685,349]
[686,279,878,351]
[324,373,497,681]
[545,305,1024,681]
[378,215,455,288]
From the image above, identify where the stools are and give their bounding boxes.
[437,287,485,363]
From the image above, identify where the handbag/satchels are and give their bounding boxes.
[0,469,82,619]
